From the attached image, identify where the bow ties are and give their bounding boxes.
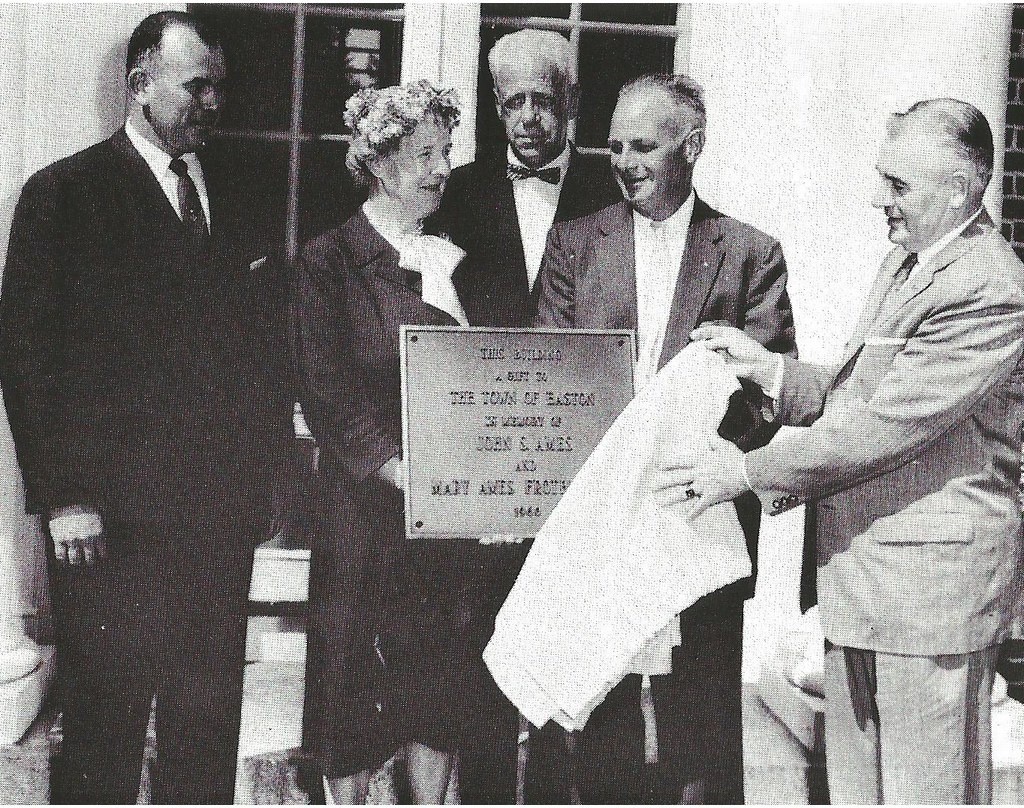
[506,164,562,185]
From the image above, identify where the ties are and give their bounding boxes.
[168,157,211,252]
[871,251,919,325]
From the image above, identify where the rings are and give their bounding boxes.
[685,488,695,499]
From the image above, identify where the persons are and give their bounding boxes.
[287,79,526,806]
[414,31,614,804]
[0,8,296,806]
[646,95,1024,805]
[521,70,806,804]
